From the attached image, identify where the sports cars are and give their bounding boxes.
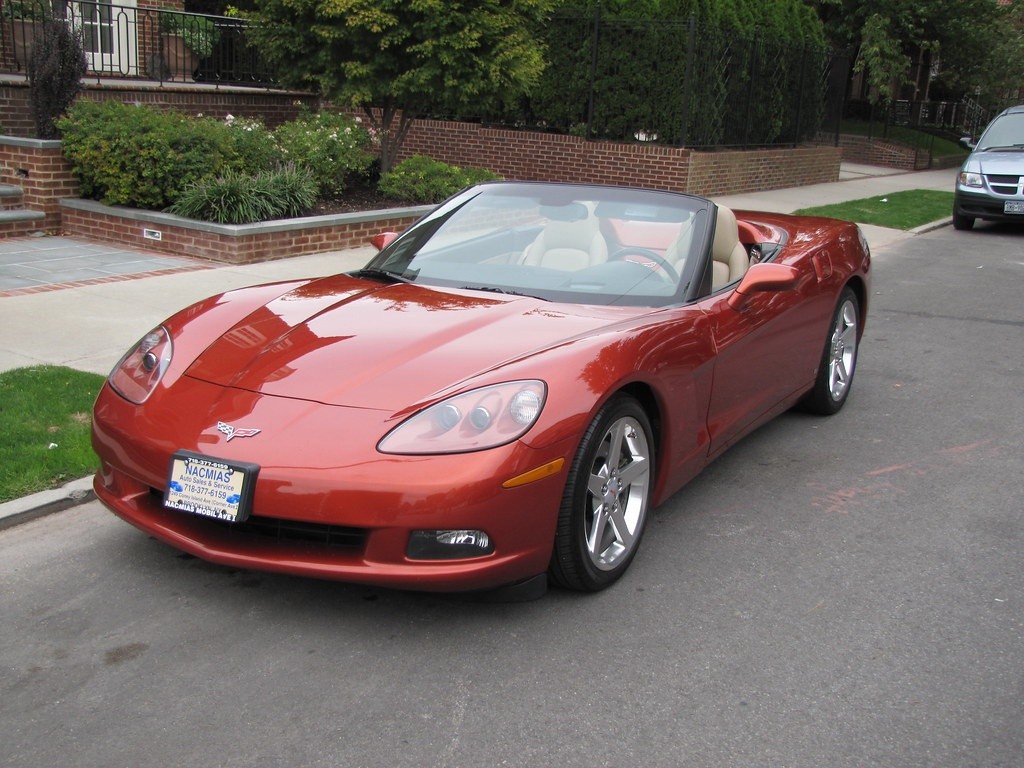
[85,181,873,605]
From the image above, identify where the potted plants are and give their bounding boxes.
[0,0,56,76]
[157,5,223,83]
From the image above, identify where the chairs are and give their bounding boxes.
[659,204,748,291]
[523,200,609,272]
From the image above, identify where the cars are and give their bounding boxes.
[951,105,1024,231]
